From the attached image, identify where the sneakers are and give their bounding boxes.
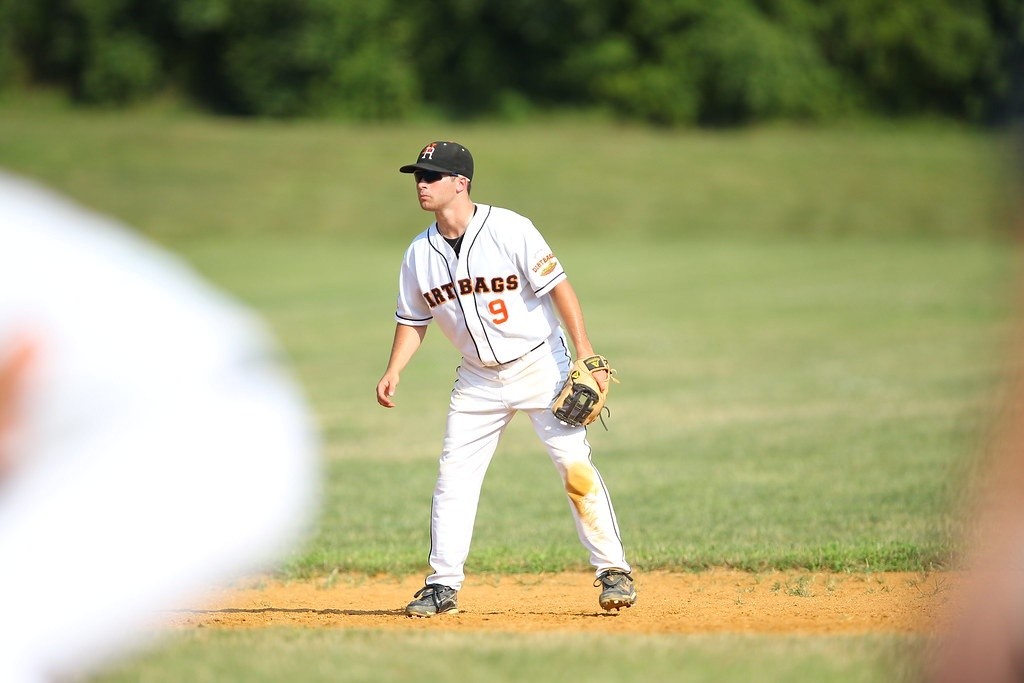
[598,571,636,611]
[405,583,459,616]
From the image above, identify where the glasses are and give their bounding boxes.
[413,169,456,182]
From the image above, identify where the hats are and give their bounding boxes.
[398,140,473,183]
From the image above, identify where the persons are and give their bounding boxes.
[374,139,637,619]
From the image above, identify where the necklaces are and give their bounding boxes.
[437,204,475,250]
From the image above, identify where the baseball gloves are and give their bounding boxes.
[551,355,620,431]
